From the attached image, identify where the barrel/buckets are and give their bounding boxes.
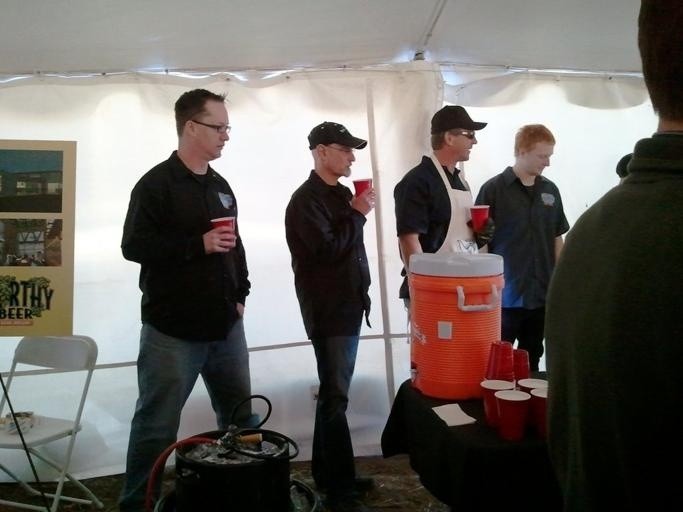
[408,253,505,400]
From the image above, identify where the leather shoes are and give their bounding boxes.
[352,477,377,491]
[323,490,371,512]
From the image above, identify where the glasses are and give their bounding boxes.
[191,119,230,134]
[458,129,474,139]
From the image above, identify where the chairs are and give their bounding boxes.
[0,337,105,509]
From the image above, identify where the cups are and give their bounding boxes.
[352,177,372,201]
[469,205,490,233]
[210,216,237,249]
[481,338,549,442]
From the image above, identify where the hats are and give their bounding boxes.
[308,121,367,150]
[430,105,487,134]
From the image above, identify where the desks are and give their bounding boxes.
[401,372,561,512]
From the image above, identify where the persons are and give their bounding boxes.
[379,103,497,343]
[473,122,572,375]
[542,0,683,512]
[113,89,254,511]
[284,120,378,511]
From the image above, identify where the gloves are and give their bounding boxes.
[466,217,495,249]
